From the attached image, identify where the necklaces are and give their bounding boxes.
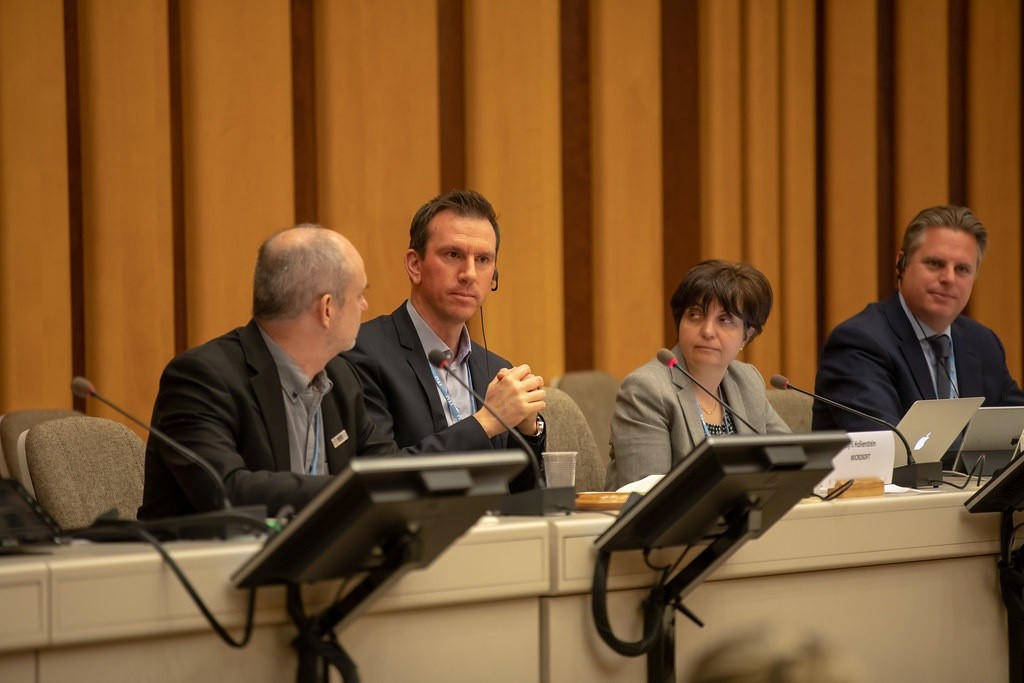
[696,385,730,437]
[309,410,320,474]
[428,360,476,422]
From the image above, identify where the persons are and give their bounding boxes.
[338,187,547,494]
[810,205,1024,432]
[604,259,793,488]
[143,222,404,519]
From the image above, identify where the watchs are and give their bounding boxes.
[532,415,544,436]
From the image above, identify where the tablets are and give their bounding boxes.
[952,406,1024,473]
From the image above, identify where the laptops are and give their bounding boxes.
[892,396,986,468]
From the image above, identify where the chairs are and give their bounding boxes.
[539,389,605,493]
[18,418,145,529]
[554,372,618,478]
[0,409,86,499]
[766,390,815,435]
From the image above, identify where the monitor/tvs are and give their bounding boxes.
[965,451,1024,513]
[232,449,530,591]
[593,430,849,553]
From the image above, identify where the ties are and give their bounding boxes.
[925,330,956,400]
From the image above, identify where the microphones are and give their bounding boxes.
[656,348,760,435]
[429,348,578,517]
[769,374,946,489]
[70,376,269,542]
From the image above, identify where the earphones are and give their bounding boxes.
[896,255,906,271]
[493,268,498,281]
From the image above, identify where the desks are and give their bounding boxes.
[0,472,1024,683]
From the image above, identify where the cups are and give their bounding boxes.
[541,451,578,517]
